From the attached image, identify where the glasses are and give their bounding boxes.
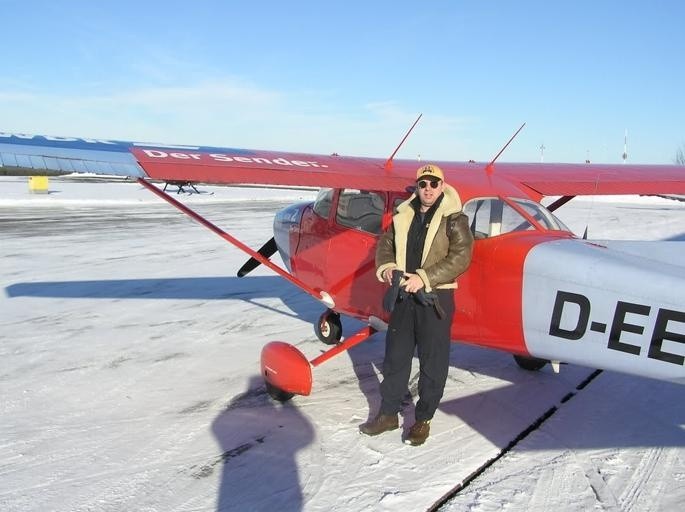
[418,181,438,188]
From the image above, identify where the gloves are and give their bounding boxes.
[384,270,409,313]
[410,287,435,307]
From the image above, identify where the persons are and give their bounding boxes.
[358,163,473,446]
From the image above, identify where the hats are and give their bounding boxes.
[416,165,444,181]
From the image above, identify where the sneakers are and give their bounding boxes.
[406,420,430,446]
[362,415,398,435]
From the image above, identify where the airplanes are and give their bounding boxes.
[0,107,685,408]
[162,180,213,195]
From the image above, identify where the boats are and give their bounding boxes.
[55,170,162,183]
